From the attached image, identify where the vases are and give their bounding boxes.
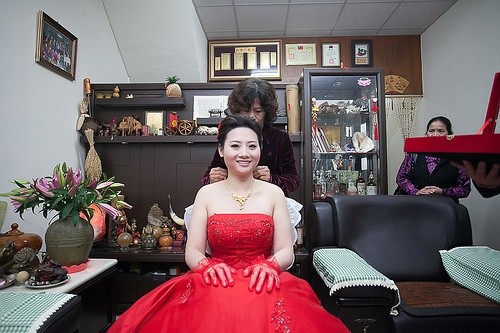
[45,217,94,267]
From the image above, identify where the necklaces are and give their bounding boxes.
[224,178,256,211]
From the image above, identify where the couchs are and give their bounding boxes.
[0,291,83,333]
[306,193,500,333]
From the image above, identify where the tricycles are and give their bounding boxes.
[165,112,195,136]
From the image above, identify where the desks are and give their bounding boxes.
[0,259,119,333]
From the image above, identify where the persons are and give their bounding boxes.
[202,77,299,197]
[106,114,352,333]
[42,37,71,71]
[393,115,471,203]
[451,159,500,198]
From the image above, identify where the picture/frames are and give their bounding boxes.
[208,40,281,82]
[351,39,373,67]
[284,43,317,66]
[35,10,79,81]
[321,42,340,68]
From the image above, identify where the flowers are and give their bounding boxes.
[0,163,133,228]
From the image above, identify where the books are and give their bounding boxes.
[312,126,331,153]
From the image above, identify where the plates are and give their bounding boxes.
[25,275,71,288]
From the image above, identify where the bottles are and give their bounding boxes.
[313,165,377,200]
[158,228,173,248]
[141,233,157,251]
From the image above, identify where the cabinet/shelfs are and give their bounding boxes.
[90,248,311,315]
[300,68,387,202]
[83,78,300,144]
